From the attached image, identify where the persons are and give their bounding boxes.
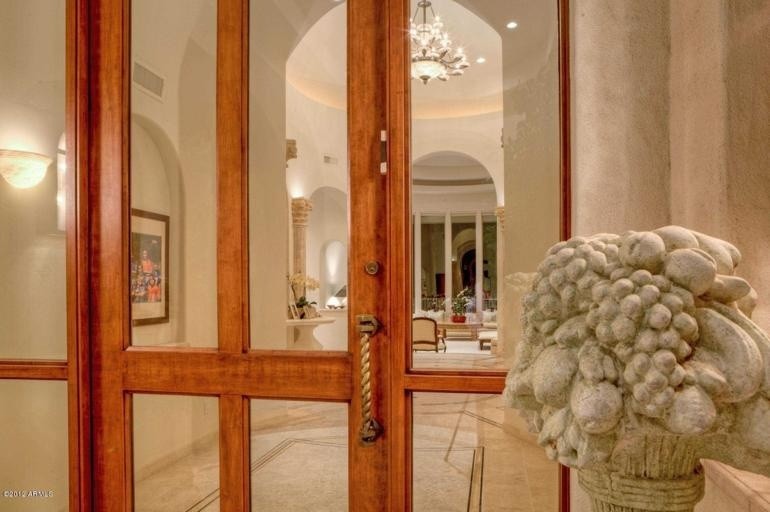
[131,250,161,302]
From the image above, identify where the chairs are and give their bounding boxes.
[412,317,448,354]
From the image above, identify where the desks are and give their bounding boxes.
[289,319,337,351]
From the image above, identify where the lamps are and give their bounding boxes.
[410,1,471,85]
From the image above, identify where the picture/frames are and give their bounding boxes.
[130,204,171,328]
[287,301,312,319]
